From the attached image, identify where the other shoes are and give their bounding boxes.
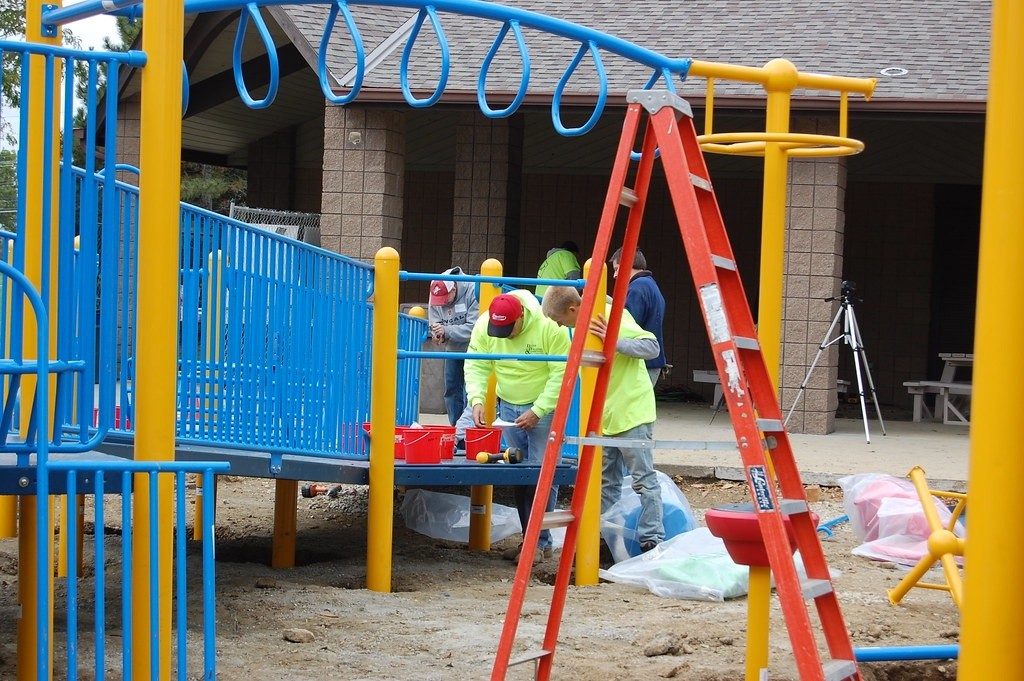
[515,549,544,565]
[518,543,553,557]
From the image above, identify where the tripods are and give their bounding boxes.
[784,294,887,445]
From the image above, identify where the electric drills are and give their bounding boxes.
[301,483,342,498]
[475,446,523,464]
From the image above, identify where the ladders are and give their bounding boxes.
[489,89,860,681]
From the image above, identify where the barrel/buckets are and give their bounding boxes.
[465,428,502,460]
[403,430,442,464]
[423,425,456,459]
[394,426,411,459]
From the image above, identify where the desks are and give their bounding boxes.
[934,352,974,421]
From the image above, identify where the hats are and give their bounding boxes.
[487,294,522,338]
[429,280,455,306]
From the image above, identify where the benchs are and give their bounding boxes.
[903,380,972,425]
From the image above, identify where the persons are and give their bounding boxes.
[535,240,582,327]
[463,290,572,567]
[609,245,672,478]
[542,284,666,566]
[428,266,479,456]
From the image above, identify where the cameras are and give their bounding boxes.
[841,280,857,295]
